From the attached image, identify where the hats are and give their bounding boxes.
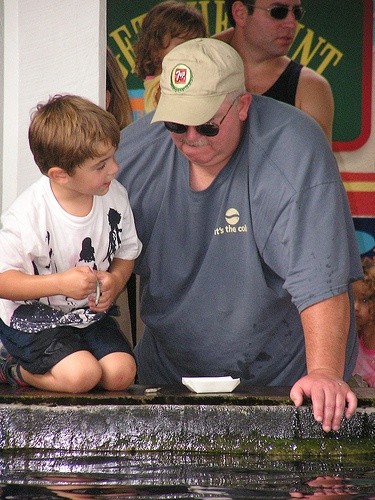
[149,37,245,128]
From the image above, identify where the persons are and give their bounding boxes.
[132,0,208,115]
[114,37,364,433]
[0,93,143,394]
[209,0,335,151]
[351,245,375,388]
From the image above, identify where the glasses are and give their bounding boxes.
[163,94,240,137]
[245,3,306,20]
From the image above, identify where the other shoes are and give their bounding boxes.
[0,342,17,389]
